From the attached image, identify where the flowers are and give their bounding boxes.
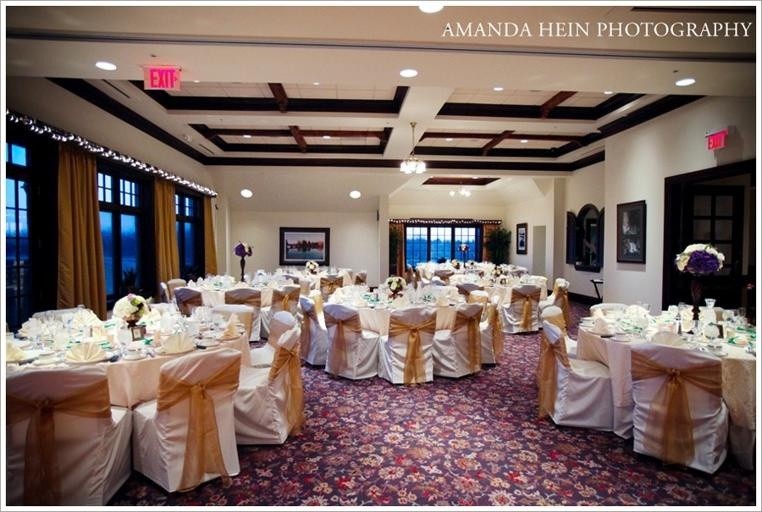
[234,241,253,257]
[676,241,725,275]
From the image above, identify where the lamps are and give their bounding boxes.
[401,121,427,176]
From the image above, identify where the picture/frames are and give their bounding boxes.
[515,223,528,255]
[279,226,331,266]
[616,199,648,266]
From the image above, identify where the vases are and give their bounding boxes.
[240,255,246,282]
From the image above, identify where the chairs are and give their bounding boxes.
[134,345,246,497]
[540,316,615,433]
[159,282,170,303]
[244,310,298,372]
[214,304,249,330]
[589,302,626,317]
[629,344,732,476]
[174,286,202,316]
[435,304,485,379]
[6,372,134,506]
[541,309,579,357]
[224,289,263,343]
[323,303,380,380]
[320,274,342,296]
[509,283,542,334]
[299,292,328,365]
[270,286,302,320]
[167,277,189,300]
[380,304,438,387]
[477,292,502,378]
[538,277,571,325]
[233,327,305,448]
[354,271,368,287]
